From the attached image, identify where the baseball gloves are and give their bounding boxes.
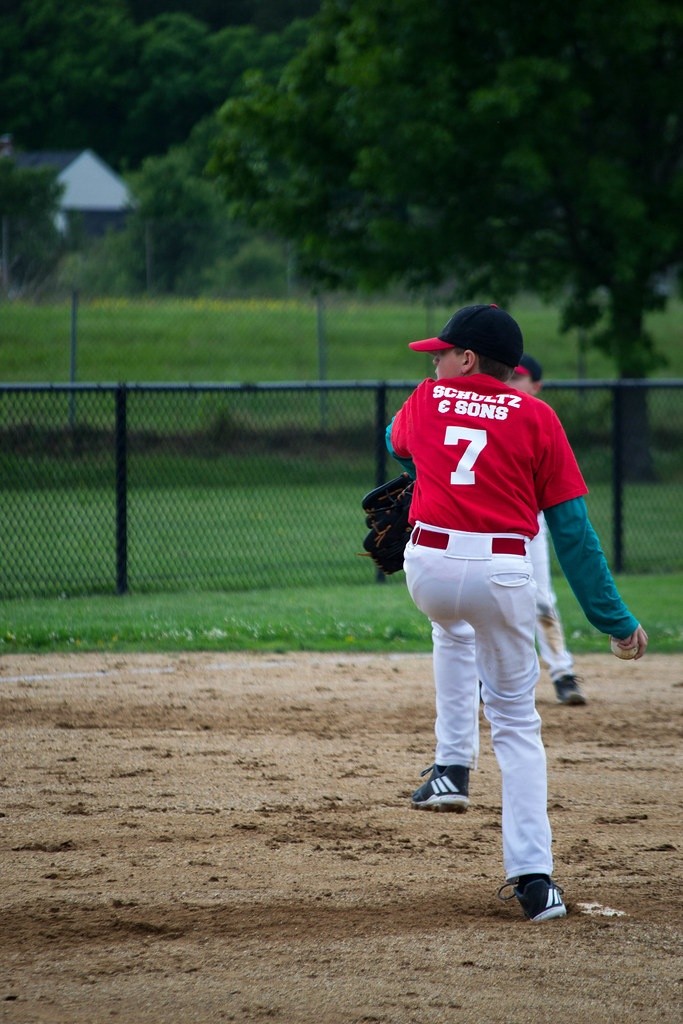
[362,473,413,575]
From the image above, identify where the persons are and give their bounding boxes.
[385,304,649,922]
[504,355,588,704]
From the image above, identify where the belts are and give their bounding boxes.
[413,527,527,556]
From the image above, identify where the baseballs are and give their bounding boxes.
[609,636,639,659]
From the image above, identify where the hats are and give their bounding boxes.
[408,303,523,367]
[515,354,541,382]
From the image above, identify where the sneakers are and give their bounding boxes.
[553,675,586,704]
[411,763,470,809]
[498,877,566,922]
